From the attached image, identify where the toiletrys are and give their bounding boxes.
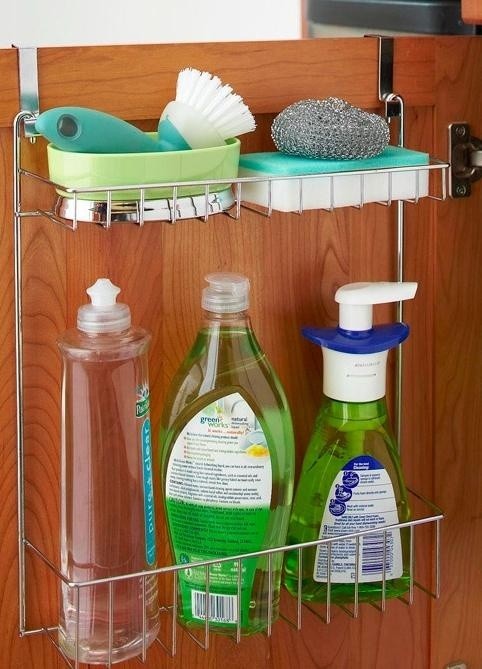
[284,279,418,600]
